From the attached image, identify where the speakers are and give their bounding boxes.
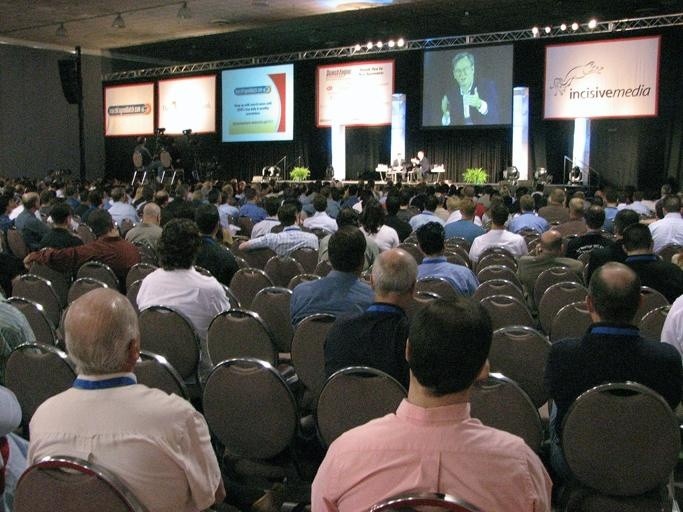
[58,60,83,104]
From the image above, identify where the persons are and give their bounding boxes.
[544,259,682,480]
[438,51,498,125]
[25,287,226,510]
[0,150,683,387]
[0,383,31,511]
[309,293,555,511]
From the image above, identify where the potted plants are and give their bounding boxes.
[289,165,311,180]
[462,168,487,181]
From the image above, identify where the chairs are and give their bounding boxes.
[365,488,477,512]
[559,385,678,512]
[202,358,309,491]
[314,364,407,450]
[13,457,147,511]
[5,341,75,434]
[468,374,542,452]
[0,187,682,405]
[376,163,446,185]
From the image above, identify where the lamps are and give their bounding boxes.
[501,167,516,182]
[262,165,280,177]
[534,167,550,182]
[54,3,194,39]
